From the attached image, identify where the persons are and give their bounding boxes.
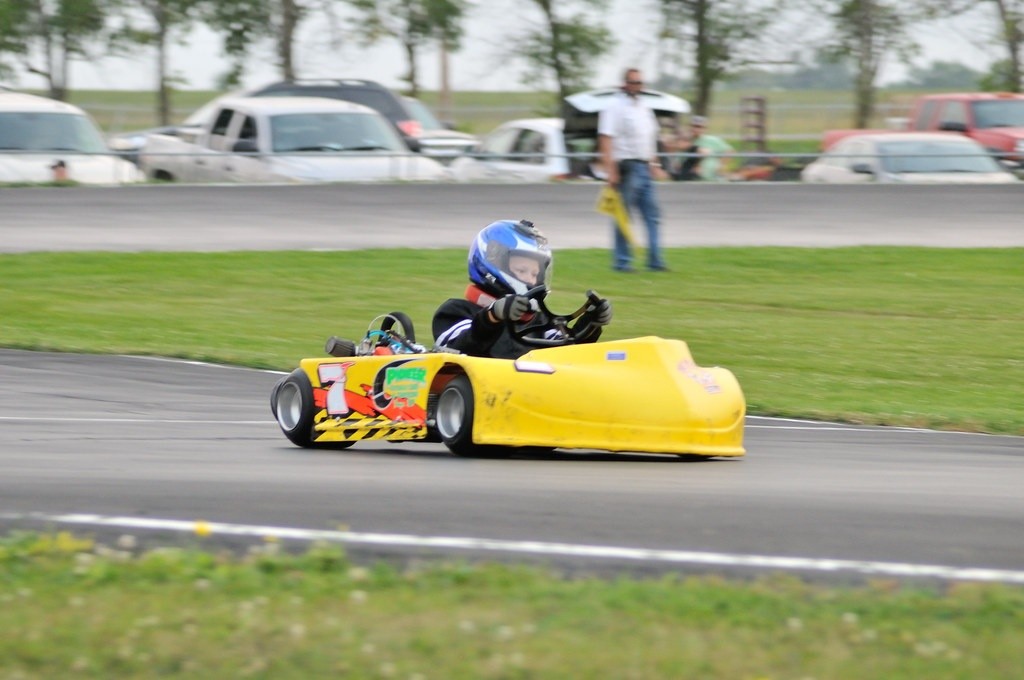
[433,221,612,357]
[657,115,733,179]
[598,68,669,272]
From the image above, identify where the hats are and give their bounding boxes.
[691,116,707,127]
[52,160,65,167]
[659,117,678,127]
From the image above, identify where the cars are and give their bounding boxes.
[449,87,691,184]
[401,96,478,157]
[800,134,1021,183]
[0,90,146,184]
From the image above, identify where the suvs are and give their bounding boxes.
[110,78,422,165]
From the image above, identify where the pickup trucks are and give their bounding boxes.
[822,92,1024,163]
[140,96,448,182]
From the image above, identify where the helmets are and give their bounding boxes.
[468,220,552,300]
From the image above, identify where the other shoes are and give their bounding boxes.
[648,265,668,271]
[616,264,634,274]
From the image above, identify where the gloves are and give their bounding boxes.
[491,296,527,319]
[589,298,613,326]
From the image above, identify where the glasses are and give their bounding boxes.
[629,81,641,85]
